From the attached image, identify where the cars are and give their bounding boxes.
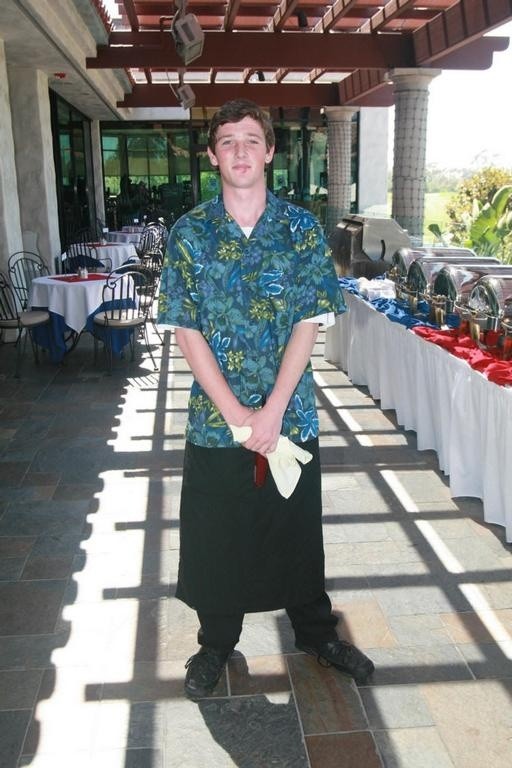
[99,236,107,245]
[78,266,88,278]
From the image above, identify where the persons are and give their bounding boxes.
[155,97,375,700]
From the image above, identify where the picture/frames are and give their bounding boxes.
[323,276,512,545]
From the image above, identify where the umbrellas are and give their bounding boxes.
[184,645,237,698]
[295,637,376,681]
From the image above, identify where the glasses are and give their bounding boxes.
[177,83,197,111]
[172,0,206,66]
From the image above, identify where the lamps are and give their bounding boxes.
[0,217,169,376]
